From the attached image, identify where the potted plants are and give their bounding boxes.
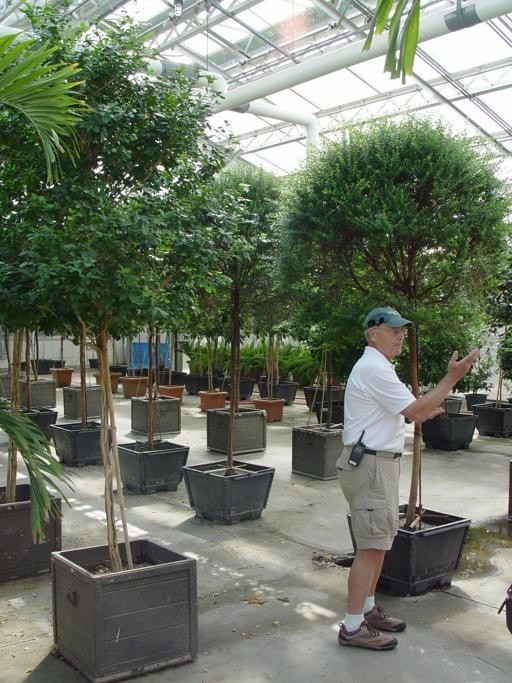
[2,2,512,683]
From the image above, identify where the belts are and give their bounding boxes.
[365,449,402,458]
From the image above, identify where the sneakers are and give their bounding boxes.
[364,602,406,632]
[338,621,397,650]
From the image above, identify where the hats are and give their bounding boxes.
[362,307,414,329]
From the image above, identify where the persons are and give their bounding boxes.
[333,304,482,652]
